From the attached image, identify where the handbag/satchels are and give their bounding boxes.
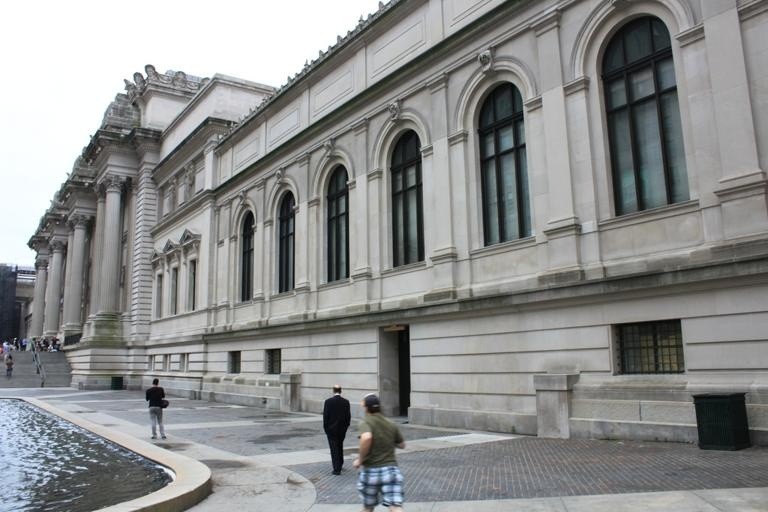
[161,400,169,408]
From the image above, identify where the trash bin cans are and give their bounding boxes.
[111,377,123,390]
[692,394,748,450]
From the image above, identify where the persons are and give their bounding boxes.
[0,333,63,380]
[321,384,351,477]
[145,378,167,439]
[350,392,405,512]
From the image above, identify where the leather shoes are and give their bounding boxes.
[332,467,342,475]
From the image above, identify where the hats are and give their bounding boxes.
[360,394,379,407]
